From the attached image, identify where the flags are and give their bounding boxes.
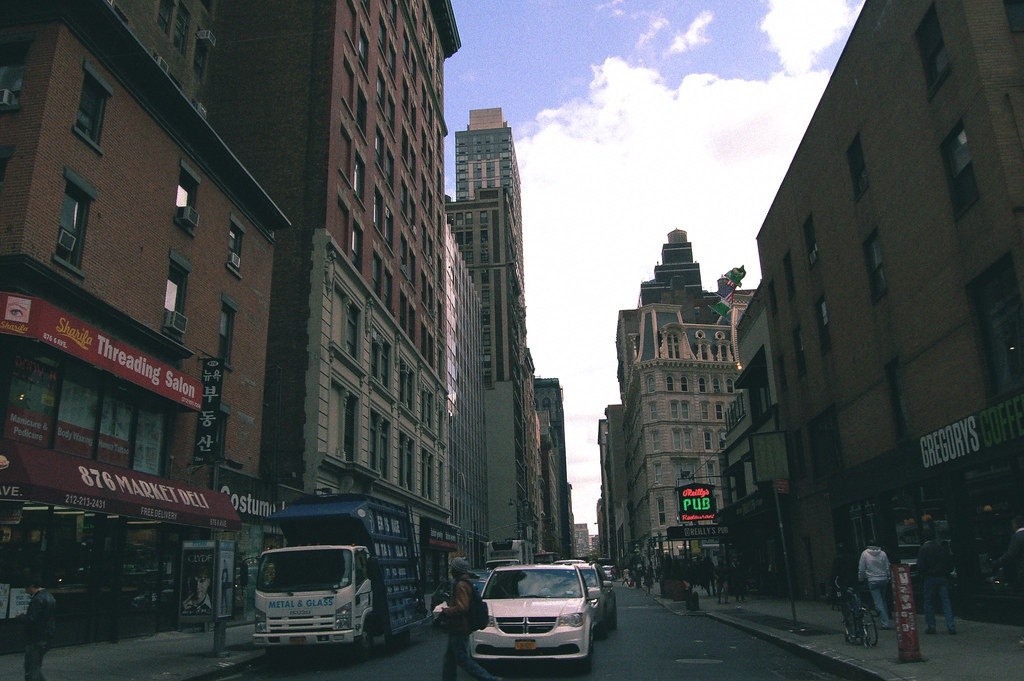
[715,280,737,303]
[708,299,733,317]
[724,264,746,286]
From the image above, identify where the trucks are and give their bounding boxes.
[485,539,536,573]
[255,494,432,661]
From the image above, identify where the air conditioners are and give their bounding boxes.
[178,206,198,227]
[155,56,169,74]
[164,310,189,336]
[0,89,16,107]
[57,231,76,251]
[197,30,216,46]
[193,102,206,119]
[228,252,242,268]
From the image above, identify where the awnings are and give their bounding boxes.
[0,438,241,532]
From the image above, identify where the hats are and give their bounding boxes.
[867,539,877,546]
[450,556,469,574]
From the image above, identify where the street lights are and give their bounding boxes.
[509,497,530,537]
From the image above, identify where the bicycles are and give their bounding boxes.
[844,587,891,647]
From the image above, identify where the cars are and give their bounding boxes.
[471,563,598,675]
[432,578,485,619]
[601,565,615,580]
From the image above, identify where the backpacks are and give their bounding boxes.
[454,580,490,631]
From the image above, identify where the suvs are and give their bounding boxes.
[537,563,618,637]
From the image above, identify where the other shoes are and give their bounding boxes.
[925,628,937,634]
[947,629,957,635]
[878,623,889,630]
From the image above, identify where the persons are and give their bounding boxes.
[622,566,644,588]
[654,554,747,604]
[181,560,211,615]
[991,515,1024,646]
[914,528,957,635]
[856,539,892,629]
[16,584,56,681]
[443,556,505,681]
